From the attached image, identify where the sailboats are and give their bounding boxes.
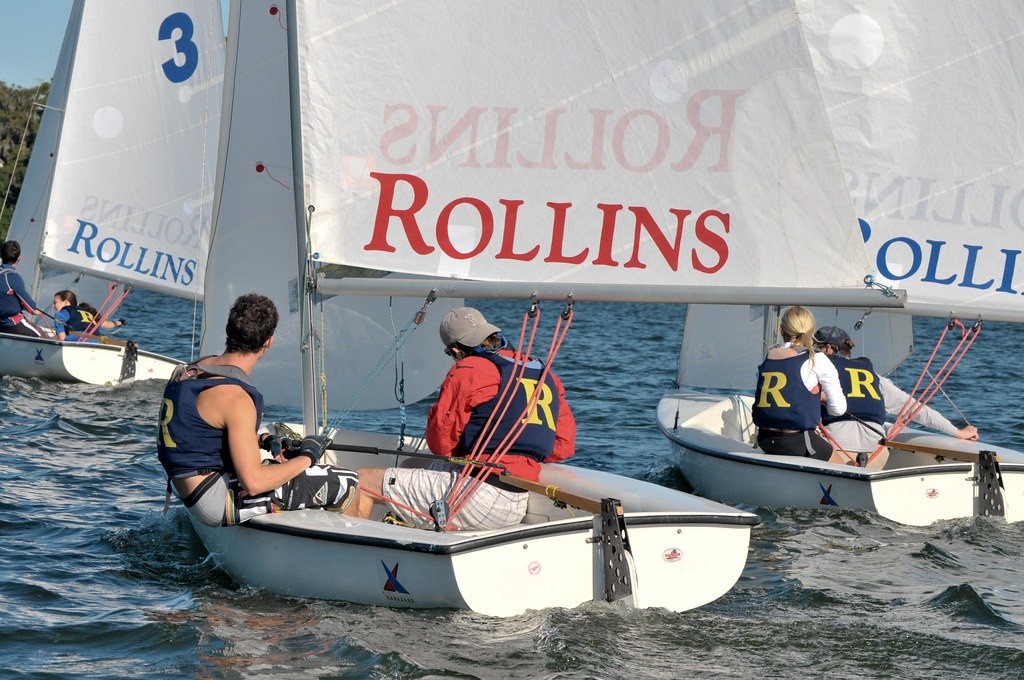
[653,0,1024,538]
[0,1,228,394]
[187,0,908,622]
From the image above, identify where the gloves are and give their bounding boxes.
[117,318,125,327]
[261,435,292,459]
[300,436,328,468]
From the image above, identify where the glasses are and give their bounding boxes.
[444,347,452,356]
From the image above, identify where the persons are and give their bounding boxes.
[752,306,979,470]
[54,290,127,343]
[0,240,42,337]
[357,307,576,531]
[157,294,360,527]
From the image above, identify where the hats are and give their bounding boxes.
[439,306,501,347]
[813,325,852,351]
